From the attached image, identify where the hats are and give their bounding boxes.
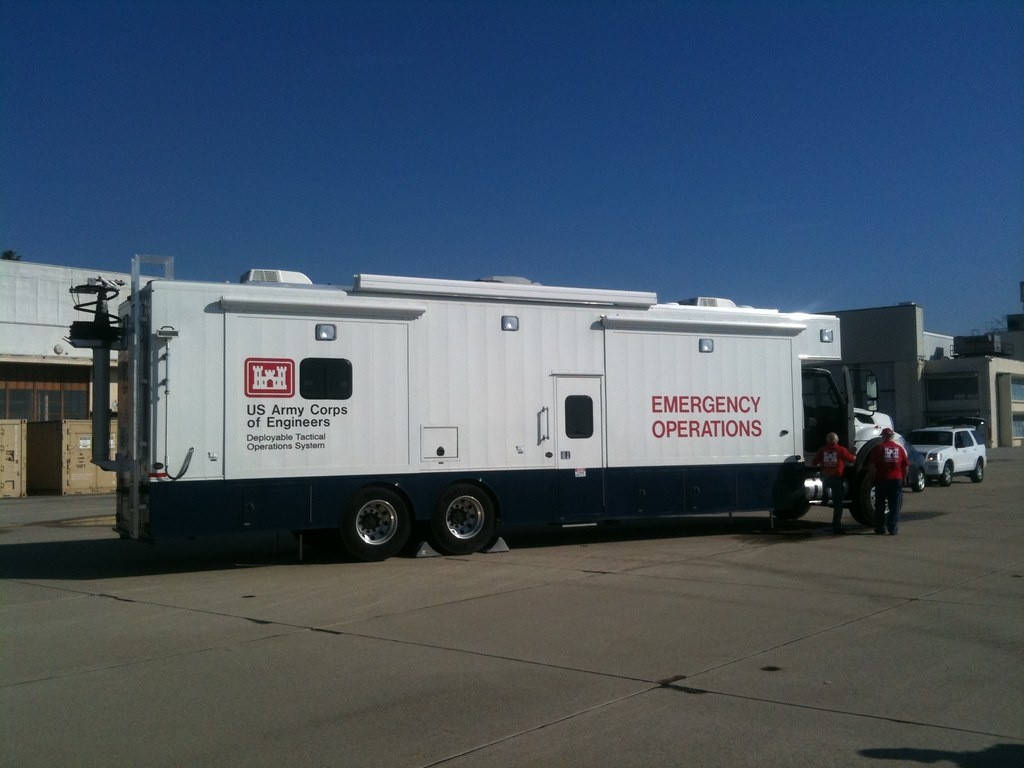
[879,428,894,436]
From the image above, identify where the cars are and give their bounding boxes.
[857,426,926,492]
[852,407,895,454]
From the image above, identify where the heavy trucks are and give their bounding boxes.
[70,257,903,558]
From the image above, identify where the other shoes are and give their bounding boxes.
[873,528,886,534]
[887,526,897,535]
[834,528,845,535]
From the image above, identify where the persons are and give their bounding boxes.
[811,432,857,530]
[869,428,910,535]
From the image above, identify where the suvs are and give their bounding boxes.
[903,426,987,487]
